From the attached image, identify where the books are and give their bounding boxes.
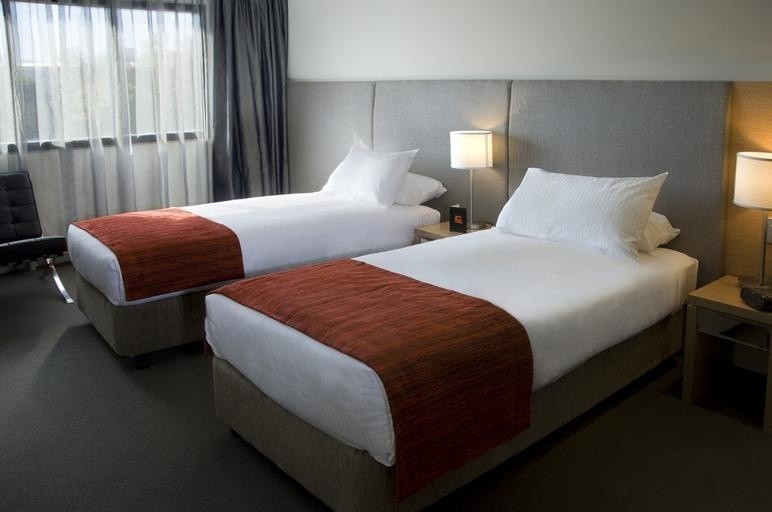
[450,203,468,233]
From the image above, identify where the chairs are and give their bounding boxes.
[0,170,74,306]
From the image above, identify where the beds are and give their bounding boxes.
[66,192,444,357]
[201,225,695,508]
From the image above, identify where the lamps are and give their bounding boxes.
[730,150,772,298]
[450,129,493,229]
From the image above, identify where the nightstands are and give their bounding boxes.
[681,277,772,432]
[411,218,490,243]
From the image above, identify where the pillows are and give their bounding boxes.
[495,168,681,265]
[320,146,448,207]
